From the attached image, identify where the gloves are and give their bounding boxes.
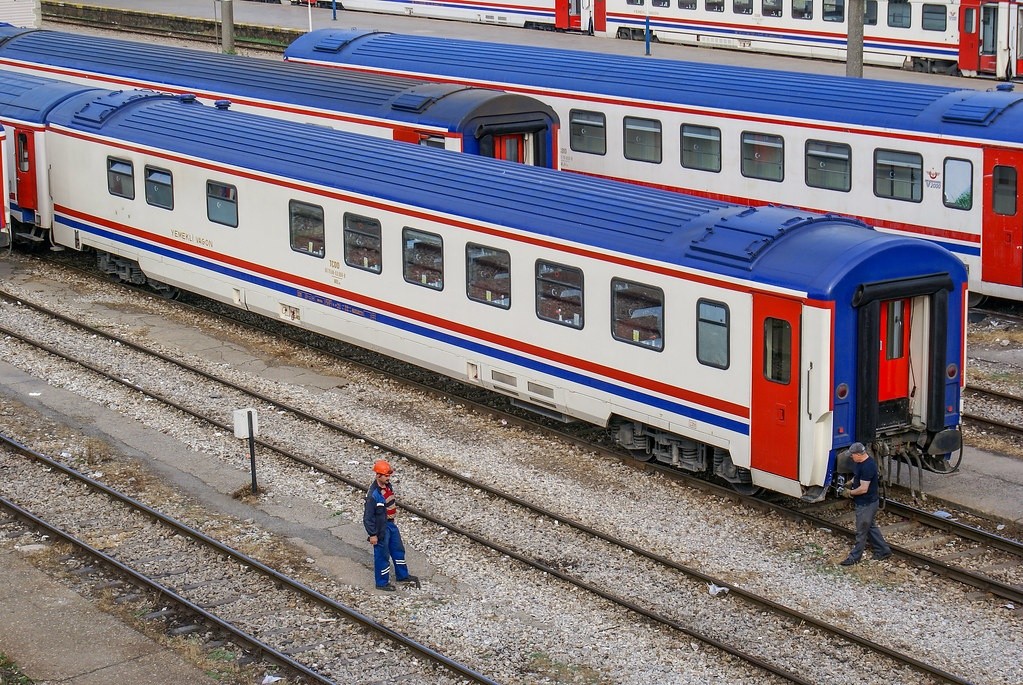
[842,481,853,490]
[839,487,855,499]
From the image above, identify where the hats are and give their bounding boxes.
[844,442,866,458]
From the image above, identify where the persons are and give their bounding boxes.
[363,461,418,591]
[627,316,662,343]
[839,442,892,566]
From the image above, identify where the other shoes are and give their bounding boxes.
[375,583,396,592]
[397,575,418,582]
[871,551,893,561]
[840,558,857,566]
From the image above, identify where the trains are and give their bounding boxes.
[253,1,1022,89]
[0,21,566,184]
[0,70,973,509]
[278,26,1022,312]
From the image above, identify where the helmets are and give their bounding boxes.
[373,462,395,476]
